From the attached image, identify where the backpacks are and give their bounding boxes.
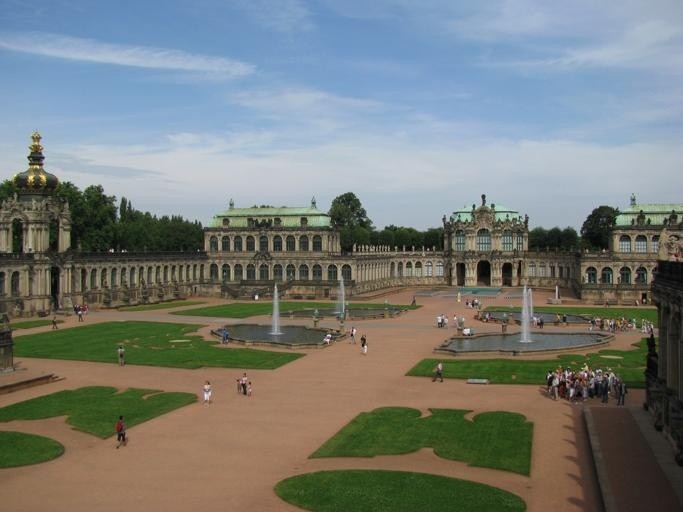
[115,422,123,433]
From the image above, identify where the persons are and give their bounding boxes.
[533,313,544,328]
[350,327,356,344]
[546,362,628,406]
[432,361,443,382]
[323,334,332,345]
[74,304,88,322]
[466,298,480,309]
[436,312,464,328]
[237,373,252,396]
[203,380,211,405]
[360,334,368,354]
[118,345,125,366]
[116,415,125,448]
[590,316,653,334]
[223,329,227,343]
[53,316,59,329]
